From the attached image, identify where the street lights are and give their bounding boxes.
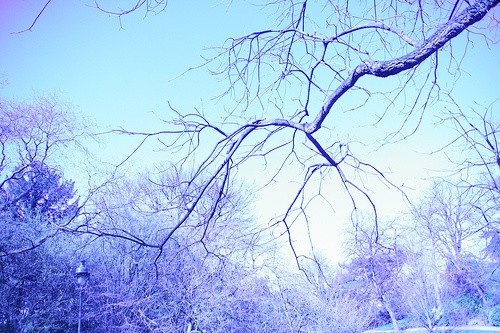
[74,259,90,333]
